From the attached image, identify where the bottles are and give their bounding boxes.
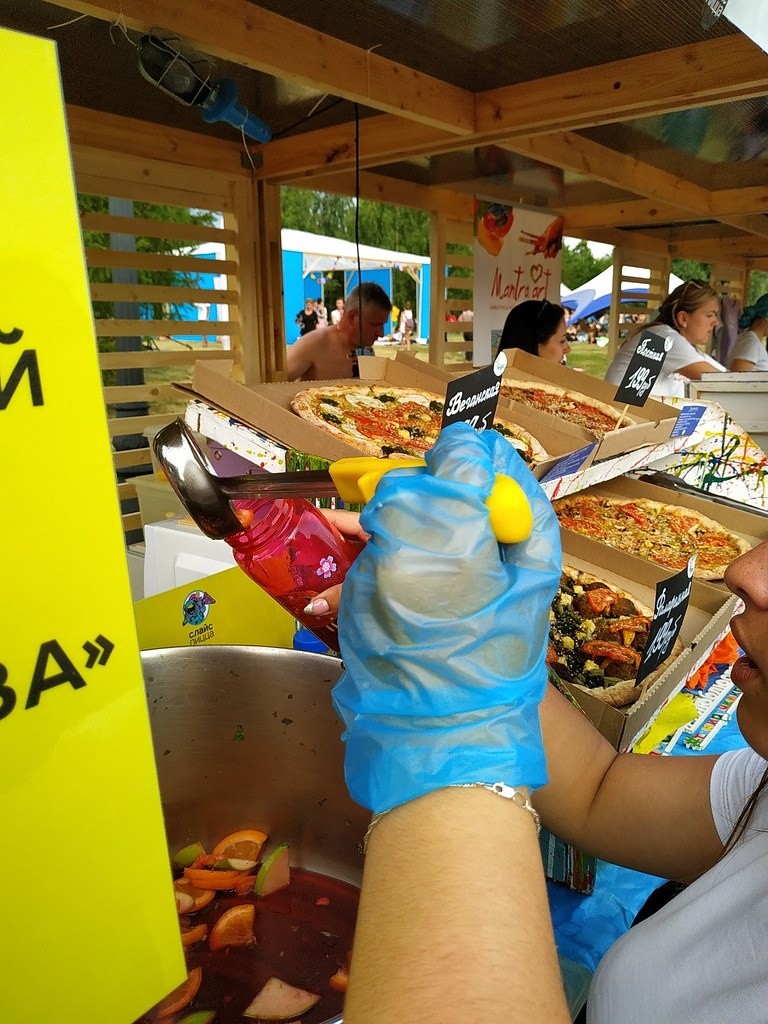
[223,497,359,652]
[293,630,331,656]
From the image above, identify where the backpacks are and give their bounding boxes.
[403,310,414,331]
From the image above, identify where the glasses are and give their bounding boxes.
[672,278,704,335]
[536,298,551,321]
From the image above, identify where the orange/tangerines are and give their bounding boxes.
[149,830,270,1019]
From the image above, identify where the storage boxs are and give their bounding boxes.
[190,347,768,748]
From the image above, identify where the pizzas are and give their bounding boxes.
[496,378,637,432]
[290,384,551,468]
[543,564,685,704]
[552,494,755,581]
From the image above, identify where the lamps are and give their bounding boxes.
[134,36,272,144]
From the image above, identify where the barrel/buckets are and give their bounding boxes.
[138,646,372,1024]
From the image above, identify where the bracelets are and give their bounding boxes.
[364,781,542,853]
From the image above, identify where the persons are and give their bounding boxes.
[296,298,329,336]
[293,421,768,1024]
[444,310,455,342]
[727,292,768,372]
[287,282,392,382]
[331,298,344,325]
[561,306,647,365]
[494,299,571,362]
[390,303,401,333]
[458,310,473,361]
[399,301,414,351]
[604,278,727,399]
